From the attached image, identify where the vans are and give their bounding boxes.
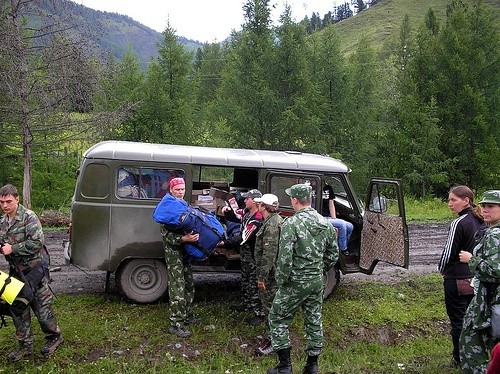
[61,139,411,304]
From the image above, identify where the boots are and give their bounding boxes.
[302,355,319,374]
[266,348,293,374]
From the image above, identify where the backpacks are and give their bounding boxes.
[0,242,33,318]
[152,193,225,261]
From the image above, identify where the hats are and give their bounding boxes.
[240,189,262,198]
[253,193,279,206]
[284,183,313,197]
[478,190,500,204]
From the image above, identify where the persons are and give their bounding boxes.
[266,184,339,374]
[438,186,485,367]
[160,178,224,337]
[0,184,63,361]
[459,190,500,374]
[222,189,284,326]
[311,180,357,257]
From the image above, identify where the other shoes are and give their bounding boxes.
[254,339,277,356]
[230,302,246,310]
[42,334,63,354]
[247,316,265,324]
[342,249,350,255]
[9,339,34,361]
[185,315,201,325]
[169,324,191,338]
